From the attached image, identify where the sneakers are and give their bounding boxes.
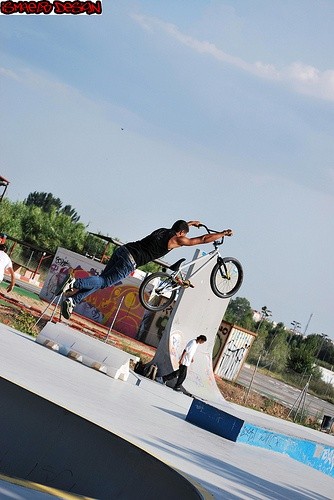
[62,297,76,319]
[54,274,76,296]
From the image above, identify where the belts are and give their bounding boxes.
[121,245,136,270]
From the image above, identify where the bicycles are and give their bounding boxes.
[139,224,244,313]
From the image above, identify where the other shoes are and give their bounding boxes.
[173,385,183,393]
[160,376,168,387]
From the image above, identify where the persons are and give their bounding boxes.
[0,232,15,294]
[156,335,207,395]
[55,221,233,321]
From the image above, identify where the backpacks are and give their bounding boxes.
[143,363,159,380]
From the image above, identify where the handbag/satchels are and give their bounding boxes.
[134,361,151,376]
